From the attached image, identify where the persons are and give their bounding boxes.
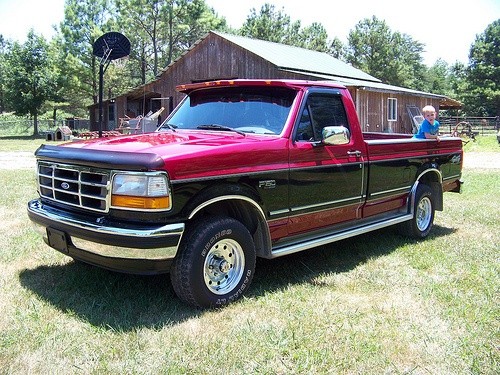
[412,105,440,139]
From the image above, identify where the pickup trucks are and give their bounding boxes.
[26,79,464,310]
